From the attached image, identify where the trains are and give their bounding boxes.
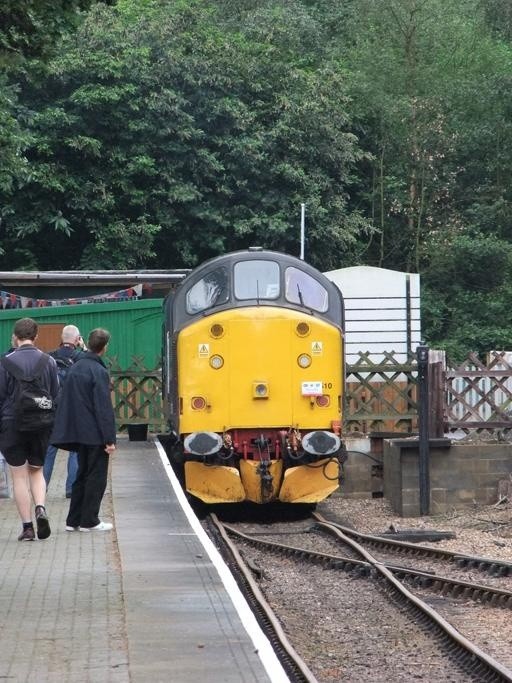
[159,241,350,524]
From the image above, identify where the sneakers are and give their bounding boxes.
[36,509,51,540]
[65,517,114,533]
[18,526,36,542]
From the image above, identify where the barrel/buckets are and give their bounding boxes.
[127,423,149,441]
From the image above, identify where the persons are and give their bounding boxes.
[0,318,92,541]
[49,329,116,532]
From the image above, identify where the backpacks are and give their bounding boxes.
[0,350,81,435]
